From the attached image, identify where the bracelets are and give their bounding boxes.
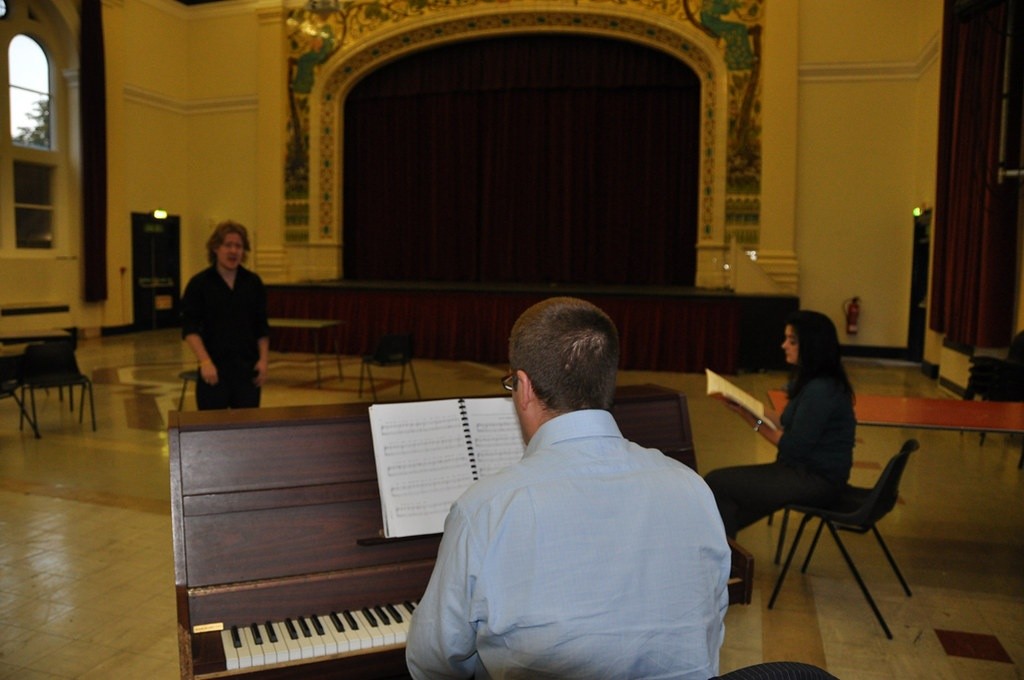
[754,419,762,433]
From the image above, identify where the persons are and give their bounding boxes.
[704,309,858,543]
[178,220,270,410]
[406,296,732,680]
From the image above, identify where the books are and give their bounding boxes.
[704,368,778,431]
[368,398,528,538]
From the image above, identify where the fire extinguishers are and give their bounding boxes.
[842,296,861,335]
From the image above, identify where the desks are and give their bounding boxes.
[767,390,1024,564]
[0,327,70,344]
[266,283,800,376]
[268,317,343,389]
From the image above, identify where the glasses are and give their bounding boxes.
[501,368,521,391]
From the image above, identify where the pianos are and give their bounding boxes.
[166,383,755,679]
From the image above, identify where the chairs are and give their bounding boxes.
[0,327,97,440]
[960,329,1024,446]
[177,369,198,413]
[359,332,421,403]
[767,438,921,640]
